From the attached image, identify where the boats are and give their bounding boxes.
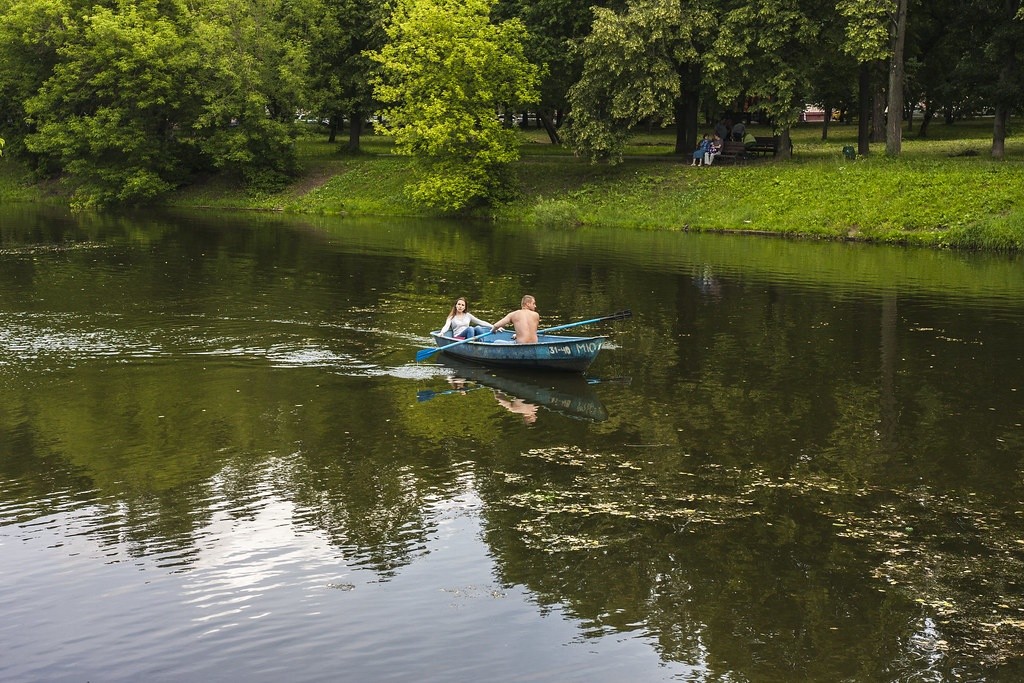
[433,353,611,425]
[429,325,610,374]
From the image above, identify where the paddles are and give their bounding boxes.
[584,377,633,385]
[511,309,634,341]
[417,385,484,403]
[417,331,494,362]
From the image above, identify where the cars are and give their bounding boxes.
[496,113,557,128]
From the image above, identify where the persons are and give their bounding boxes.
[490,295,540,344]
[437,298,505,342]
[690,117,746,167]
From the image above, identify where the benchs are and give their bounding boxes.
[748,135,794,158]
[690,142,746,162]
